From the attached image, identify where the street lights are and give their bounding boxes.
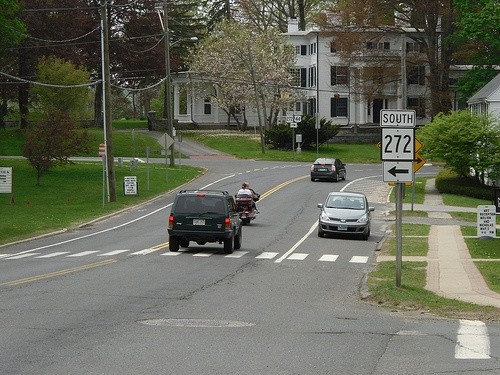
[162,1,198,168]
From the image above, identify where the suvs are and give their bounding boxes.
[167,189,242,254]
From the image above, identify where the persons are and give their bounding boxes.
[237,182,258,211]
[173,127,176,140]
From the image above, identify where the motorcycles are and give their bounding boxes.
[234,192,260,226]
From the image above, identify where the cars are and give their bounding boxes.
[310,158,346,182]
[317,191,375,241]
